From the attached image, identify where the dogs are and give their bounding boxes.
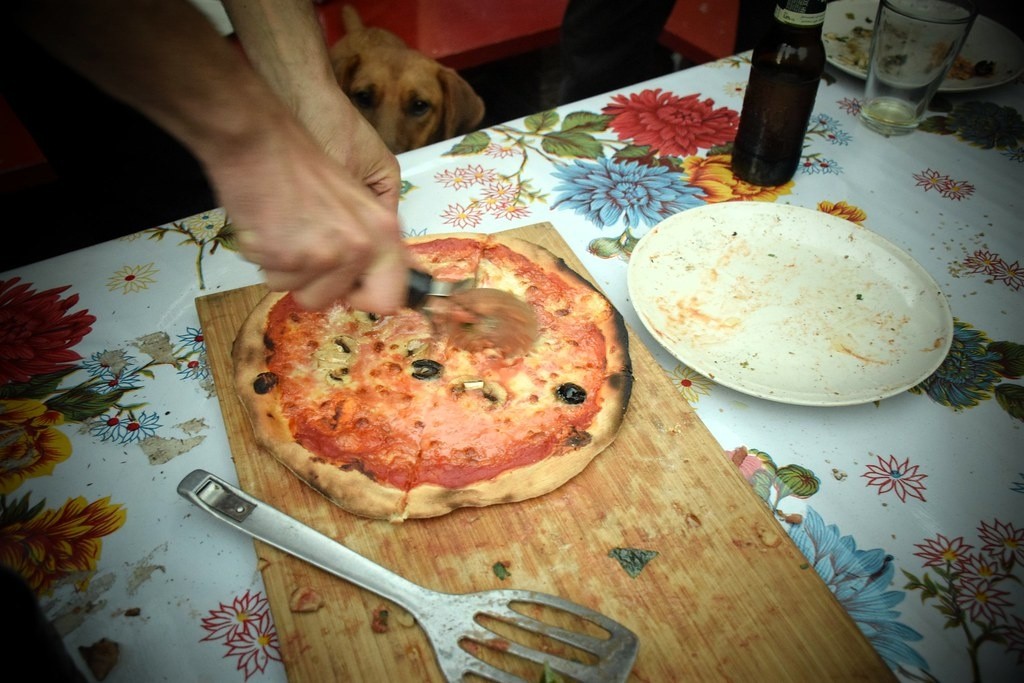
[329,2,485,159]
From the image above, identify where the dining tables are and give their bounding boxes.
[0,47,1023,682]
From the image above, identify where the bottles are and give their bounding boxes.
[730,0,828,186]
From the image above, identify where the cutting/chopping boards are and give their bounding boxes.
[193,221,896,682]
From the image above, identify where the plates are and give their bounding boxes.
[822,0,1024,95]
[627,202,954,405]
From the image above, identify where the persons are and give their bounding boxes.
[0,0,411,320]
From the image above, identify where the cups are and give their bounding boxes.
[860,0,980,136]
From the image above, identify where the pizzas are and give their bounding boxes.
[230,233,632,522]
